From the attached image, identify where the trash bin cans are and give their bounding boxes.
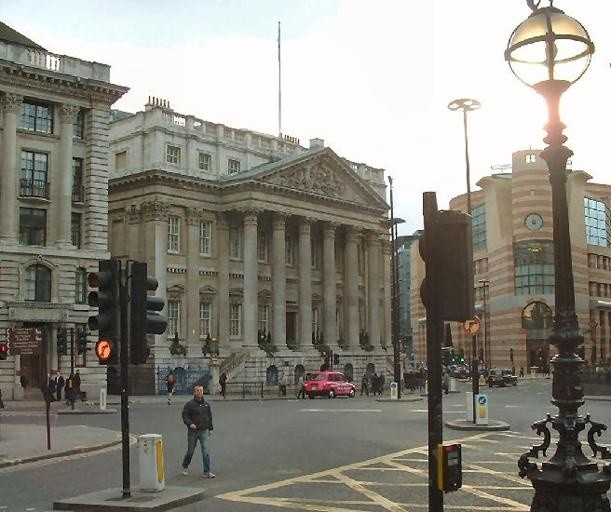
[137,434,165,492]
[531,367,539,377]
[475,394,488,424]
[390,382,398,399]
[464,392,473,422]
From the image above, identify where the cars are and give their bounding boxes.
[410,360,489,378]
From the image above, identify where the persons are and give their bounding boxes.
[180,384,217,479]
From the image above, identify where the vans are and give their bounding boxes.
[305,371,356,399]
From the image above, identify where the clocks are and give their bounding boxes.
[525,213,543,231]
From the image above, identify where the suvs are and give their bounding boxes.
[487,367,519,387]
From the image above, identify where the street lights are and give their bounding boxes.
[504,0,611,511]
[478,279,491,382]
[448,99,482,423]
[390,217,407,400]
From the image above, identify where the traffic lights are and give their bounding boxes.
[510,349,514,362]
[88,259,121,367]
[452,350,456,363]
[57,328,68,356]
[324,353,330,366]
[334,353,340,365]
[1,343,8,359]
[77,329,92,355]
[461,348,465,362]
[479,348,485,364]
[132,261,168,365]
